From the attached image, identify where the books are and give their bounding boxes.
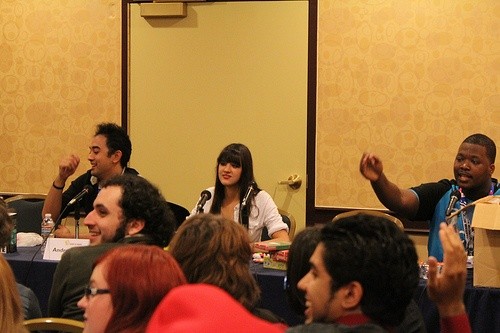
[255,240,292,251]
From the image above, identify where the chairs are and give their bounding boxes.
[23,317,84,333]
[330,209,406,233]
[260,208,297,245]
[164,202,190,231]
[4,193,49,238]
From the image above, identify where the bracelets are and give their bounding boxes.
[53,179,65,189]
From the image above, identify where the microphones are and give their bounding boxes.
[67,184,93,206]
[446,191,461,216]
[197,189,211,210]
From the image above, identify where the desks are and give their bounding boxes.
[1,243,500,332]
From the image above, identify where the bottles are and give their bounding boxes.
[41,214,55,255]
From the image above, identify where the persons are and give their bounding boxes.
[48,173,176,323]
[359,133,500,262]
[286,214,472,333]
[0,198,43,333]
[77,244,188,333]
[167,214,262,311]
[186,143,289,252]
[285,222,427,333]
[41,123,139,239]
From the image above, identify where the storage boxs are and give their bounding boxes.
[444,194,500,289]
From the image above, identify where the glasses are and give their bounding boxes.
[85,286,113,297]
